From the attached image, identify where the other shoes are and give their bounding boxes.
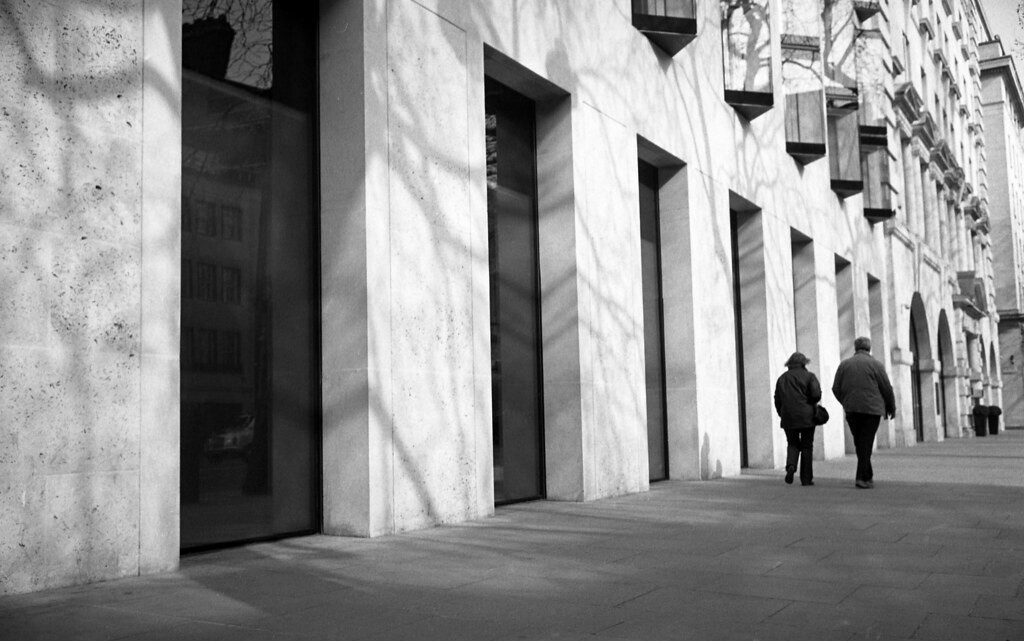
[866,479,874,487]
[856,478,868,488]
[801,479,815,486]
[785,465,796,485]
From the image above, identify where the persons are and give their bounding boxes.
[774,352,822,486]
[832,336,896,488]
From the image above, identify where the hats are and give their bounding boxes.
[784,353,810,367]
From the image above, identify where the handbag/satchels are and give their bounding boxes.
[813,402,829,425]
